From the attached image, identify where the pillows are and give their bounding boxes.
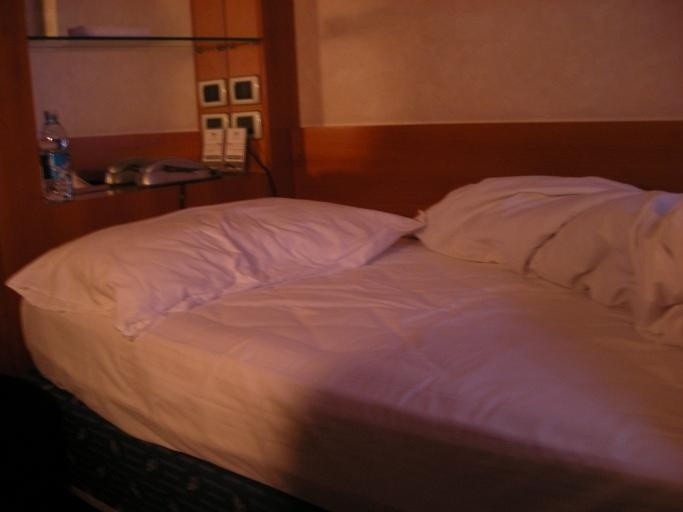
[4,197,425,342]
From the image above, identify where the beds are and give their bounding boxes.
[20,196,681,508]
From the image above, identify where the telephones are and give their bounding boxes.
[136,158,222,187]
[105,159,157,185]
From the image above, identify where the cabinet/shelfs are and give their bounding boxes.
[1,0,298,373]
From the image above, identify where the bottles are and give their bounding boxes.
[41,112,74,201]
